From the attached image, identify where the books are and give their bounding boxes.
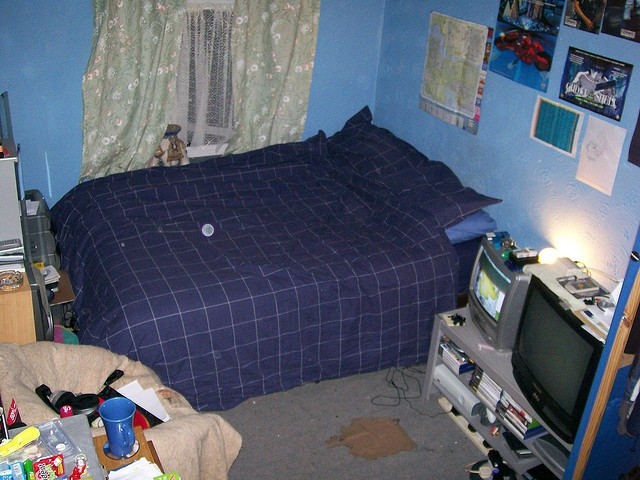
[440,343,474,375]
[495,390,550,443]
[468,364,502,412]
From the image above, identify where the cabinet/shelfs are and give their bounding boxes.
[0,138,48,342]
[421,307,574,480]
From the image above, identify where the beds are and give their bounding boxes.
[50,106,504,413]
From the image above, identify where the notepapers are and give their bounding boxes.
[443,314,466,327]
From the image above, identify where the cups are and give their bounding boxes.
[98,397,136,457]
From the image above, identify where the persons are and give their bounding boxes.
[623,0,640,22]
[566,60,615,106]
[574,0,604,32]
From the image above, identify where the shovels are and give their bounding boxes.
[92,368,130,395]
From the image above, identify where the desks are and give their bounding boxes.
[90,425,164,479]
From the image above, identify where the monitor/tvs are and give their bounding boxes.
[468,231,539,353]
[511,257,616,444]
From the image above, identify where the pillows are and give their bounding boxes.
[324,107,503,247]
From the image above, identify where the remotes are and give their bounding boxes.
[37,418,80,459]
[0,238,22,251]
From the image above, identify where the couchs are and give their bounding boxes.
[0,343,243,480]
